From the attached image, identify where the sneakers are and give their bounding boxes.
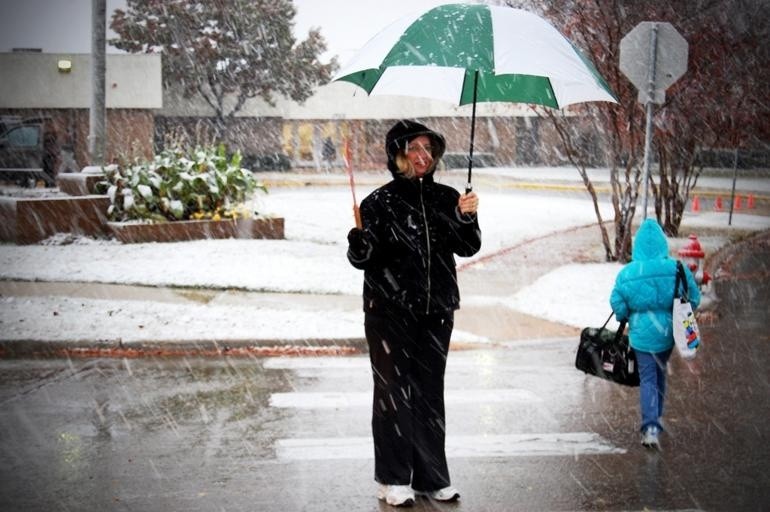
[379,484,415,507]
[416,485,460,502]
[640,423,659,447]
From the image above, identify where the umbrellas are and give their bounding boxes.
[330,3,621,195]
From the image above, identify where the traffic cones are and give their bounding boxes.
[714,196,723,211]
[748,194,755,209]
[692,195,700,213]
[735,196,742,210]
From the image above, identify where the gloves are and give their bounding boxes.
[347,227,371,260]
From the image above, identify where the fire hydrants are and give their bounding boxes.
[678,234,712,285]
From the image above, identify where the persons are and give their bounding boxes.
[43,118,58,186]
[610,218,700,448]
[348,120,481,507]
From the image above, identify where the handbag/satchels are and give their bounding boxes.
[671,298,700,361]
[575,327,640,387]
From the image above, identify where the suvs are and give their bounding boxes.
[0,120,46,176]
